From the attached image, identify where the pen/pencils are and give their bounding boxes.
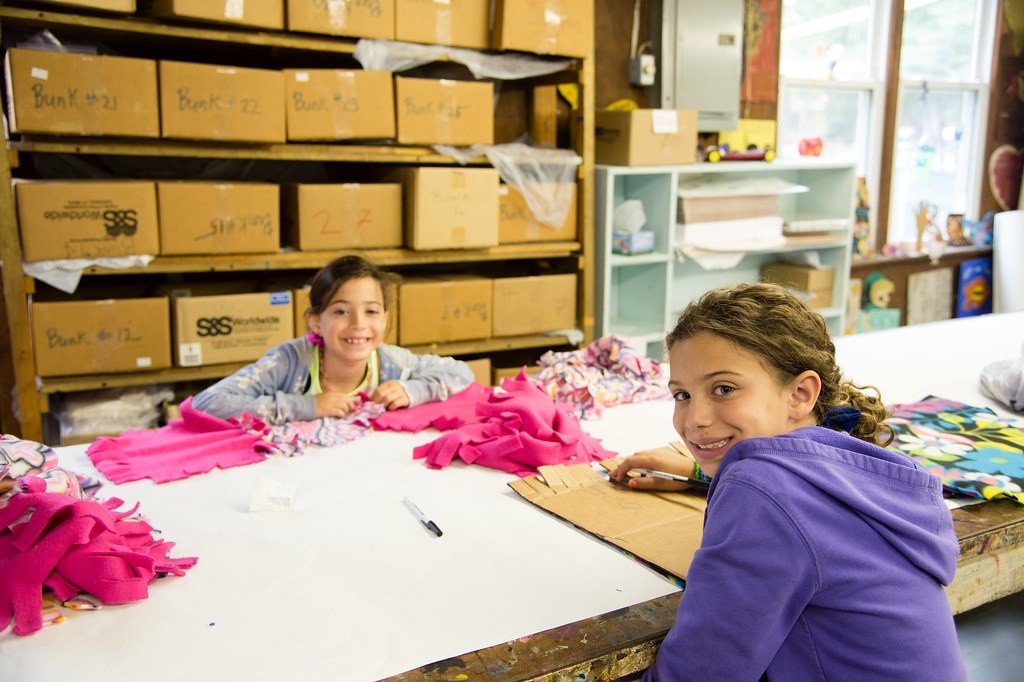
[403,496,443,538]
[617,464,711,488]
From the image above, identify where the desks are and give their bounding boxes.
[0,312,1024,682]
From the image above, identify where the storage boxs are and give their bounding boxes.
[678,193,783,221]
[397,166,501,251]
[492,272,579,340]
[174,288,294,369]
[762,265,834,292]
[702,119,777,161]
[596,107,699,166]
[294,283,399,345]
[48,0,596,60]
[287,180,402,251]
[158,180,282,258]
[160,60,286,144]
[5,49,160,138]
[805,290,833,308]
[499,180,578,245]
[13,179,160,262]
[284,68,397,145]
[32,295,173,376]
[493,365,541,385]
[393,74,498,147]
[398,276,492,346]
[60,412,158,445]
[465,356,492,386]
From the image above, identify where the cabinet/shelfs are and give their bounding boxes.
[0,0,596,443]
[597,159,859,357]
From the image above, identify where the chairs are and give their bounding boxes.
[993,210,1024,312]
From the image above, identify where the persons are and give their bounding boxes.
[611,284,968,682]
[191,255,474,426]
